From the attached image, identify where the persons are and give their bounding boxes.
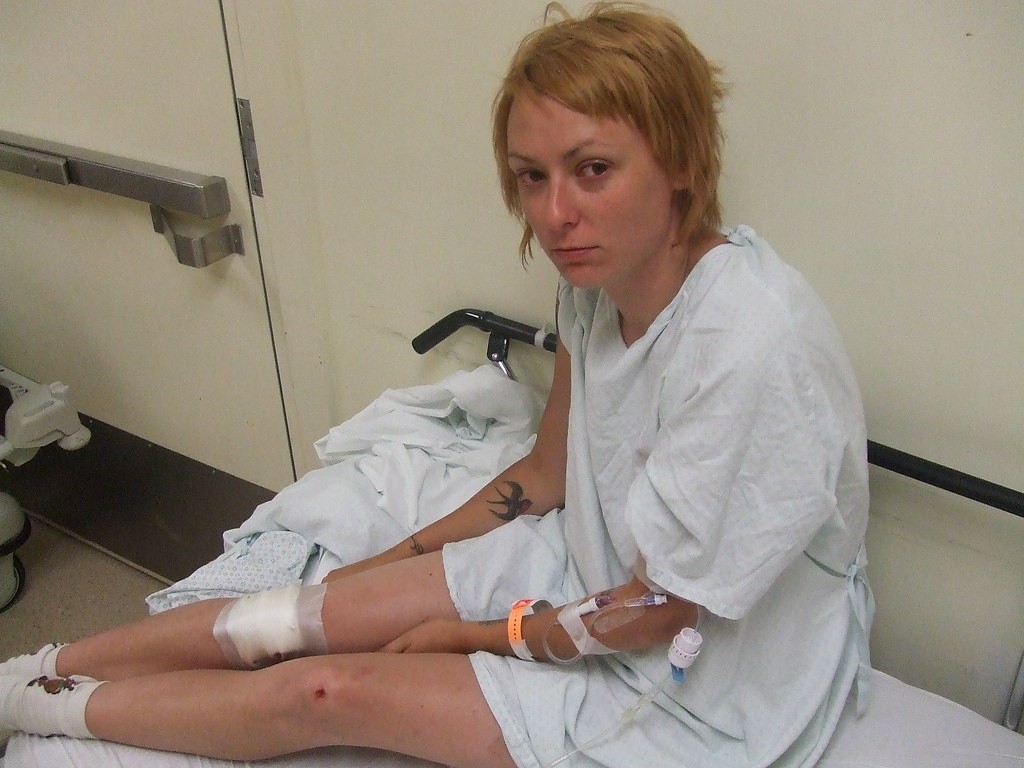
[2,4,873,768]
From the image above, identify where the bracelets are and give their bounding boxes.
[508,598,555,662]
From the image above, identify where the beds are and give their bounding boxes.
[1,310,1023,767]
